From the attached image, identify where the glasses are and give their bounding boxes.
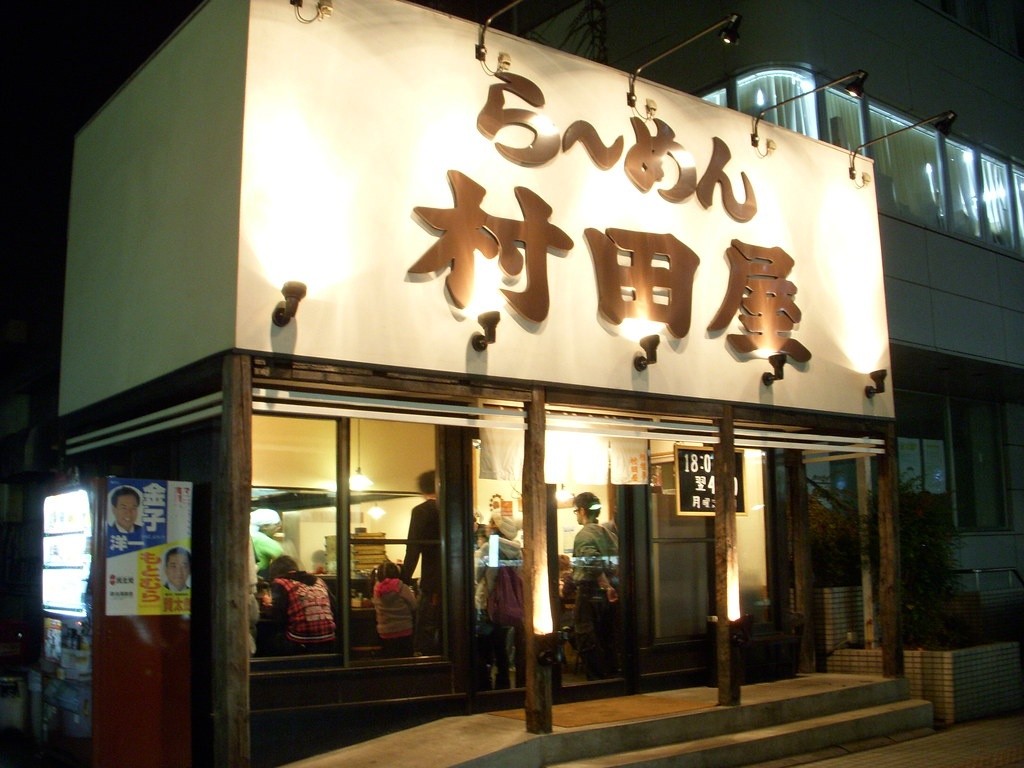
[573,509,580,515]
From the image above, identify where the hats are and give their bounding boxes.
[492,514,523,539]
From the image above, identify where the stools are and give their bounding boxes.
[351,644,386,661]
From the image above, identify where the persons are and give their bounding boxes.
[570,492,621,681]
[311,550,327,573]
[263,556,340,655]
[558,555,577,599]
[473,514,526,690]
[249,532,259,655]
[111,487,141,533]
[250,507,284,571]
[368,562,418,659]
[400,469,443,657]
[162,547,189,591]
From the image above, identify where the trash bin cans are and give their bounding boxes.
[0,677,28,733]
[707,612,753,688]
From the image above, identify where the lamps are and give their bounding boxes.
[272,281,307,328]
[762,353,787,387]
[634,335,660,371]
[865,369,887,398]
[850,110,957,189]
[471,310,500,352]
[752,69,868,158]
[349,419,373,490]
[626,14,742,121]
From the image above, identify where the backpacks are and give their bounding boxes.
[488,551,525,626]
